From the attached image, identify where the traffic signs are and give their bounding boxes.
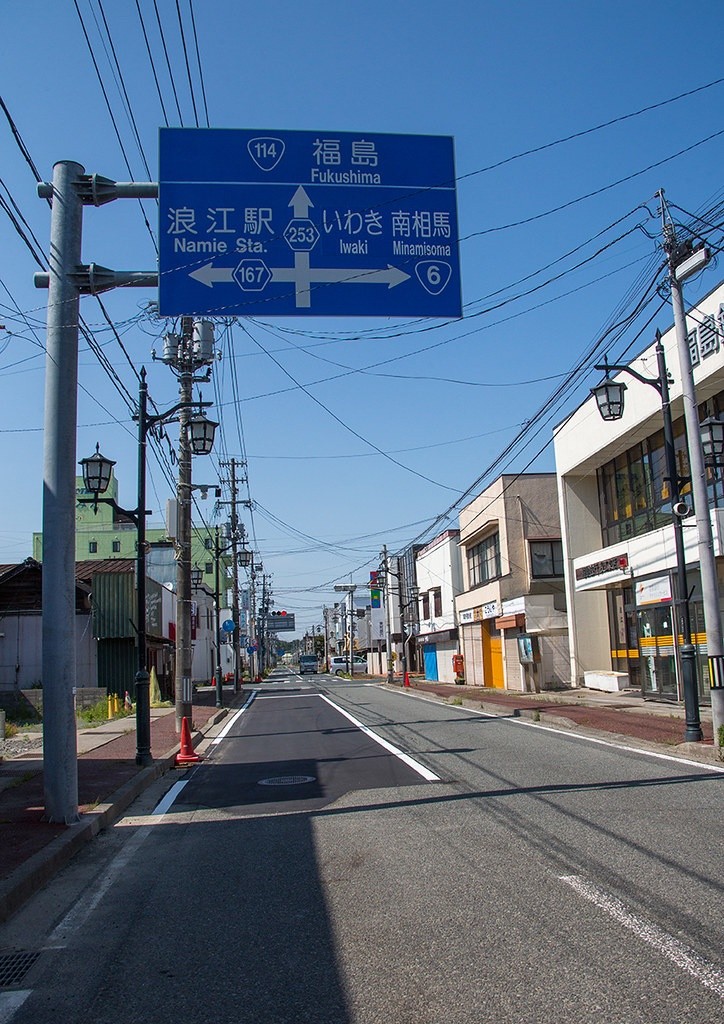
[157,125,462,319]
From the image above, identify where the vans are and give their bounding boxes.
[329,655,367,676]
[298,654,320,674]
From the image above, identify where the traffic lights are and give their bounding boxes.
[272,610,287,616]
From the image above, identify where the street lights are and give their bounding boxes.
[191,524,252,708]
[588,326,724,743]
[376,557,421,679]
[77,364,221,766]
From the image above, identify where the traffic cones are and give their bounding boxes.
[177,716,203,762]
[404,673,410,687]
[125,691,133,710]
[211,677,217,686]
